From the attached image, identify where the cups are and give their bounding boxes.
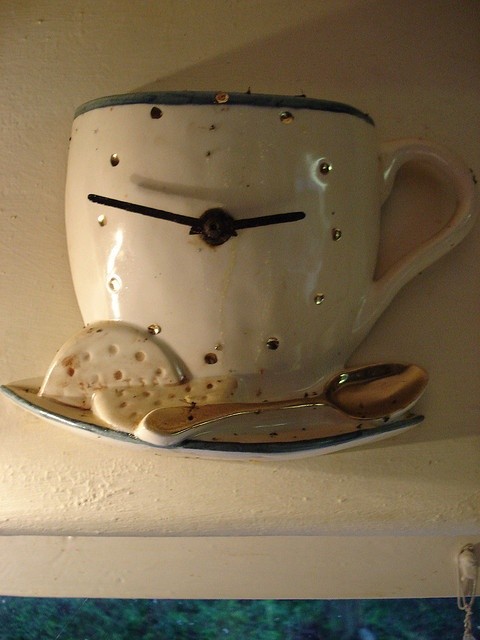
[0,91,478,464]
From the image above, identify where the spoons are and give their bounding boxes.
[133,364,430,448]
